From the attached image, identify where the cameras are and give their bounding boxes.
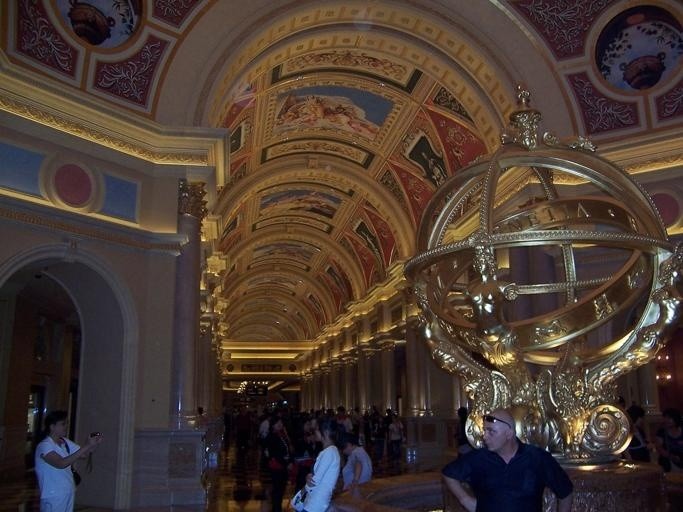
[90,432,104,438]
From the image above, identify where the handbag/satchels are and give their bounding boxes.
[290,487,310,512]
[73,471,81,486]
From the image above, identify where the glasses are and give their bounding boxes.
[483,415,511,429]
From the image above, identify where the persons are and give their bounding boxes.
[454,407,467,446]
[441,409,573,512]
[222,403,408,512]
[34,411,104,512]
[619,396,683,473]
